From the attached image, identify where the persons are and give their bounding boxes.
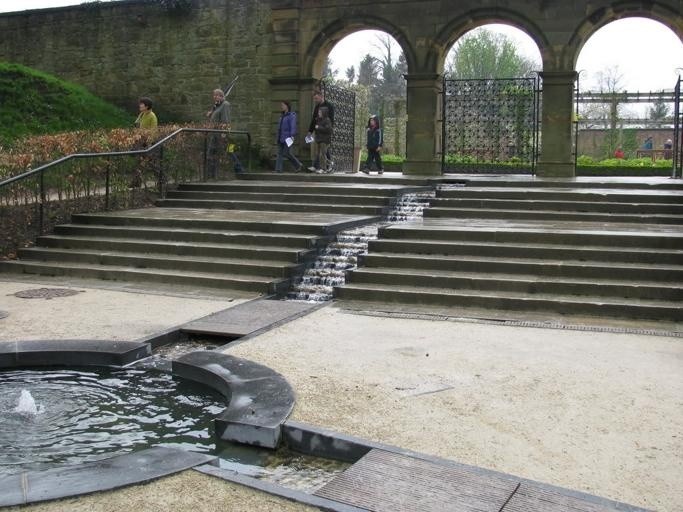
[663,139,672,157]
[203,89,244,177]
[307,92,336,173]
[639,136,653,156]
[361,115,383,175]
[128,98,168,187]
[309,107,334,174]
[270,100,305,173]
[614,146,624,159]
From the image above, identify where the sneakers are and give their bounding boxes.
[378,169,384,174]
[306,161,337,174]
[272,170,282,173]
[158,174,168,185]
[295,162,303,173]
[128,179,141,188]
[362,169,369,174]
